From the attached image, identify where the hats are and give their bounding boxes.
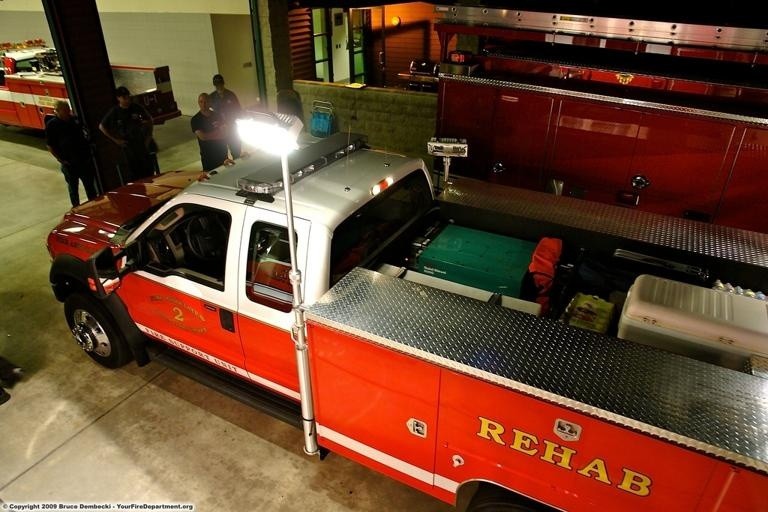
[113,85,131,97]
[213,75,224,86]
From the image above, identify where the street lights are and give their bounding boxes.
[236,111,327,464]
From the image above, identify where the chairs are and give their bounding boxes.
[309,100,336,138]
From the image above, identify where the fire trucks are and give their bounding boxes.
[0,39,182,139]
[45,126,767,511]
[390,5,767,240]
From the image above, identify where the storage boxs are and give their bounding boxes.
[616,273,766,373]
[419,224,540,300]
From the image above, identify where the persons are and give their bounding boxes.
[97,86,156,186]
[209,74,243,160]
[43,99,97,207]
[190,92,231,172]
